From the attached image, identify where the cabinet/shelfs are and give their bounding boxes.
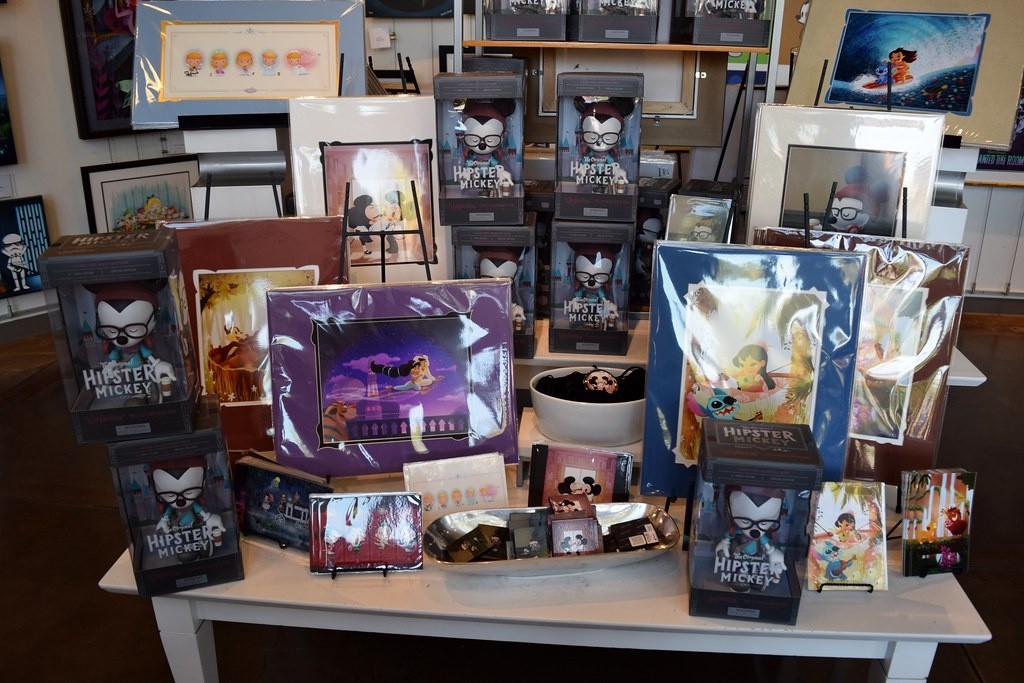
[453,0,786,320]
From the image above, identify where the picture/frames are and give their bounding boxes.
[525,48,728,147]
[58,0,144,138]
[81,154,204,233]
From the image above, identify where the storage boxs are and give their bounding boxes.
[687,418,825,626]
[548,72,645,356]
[37,227,203,445]
[670,0,776,47]
[105,394,244,597]
[523,179,743,316]
[566,0,661,43]
[484,0,568,40]
[434,58,540,360]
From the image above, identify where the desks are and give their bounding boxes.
[99,317,992,683]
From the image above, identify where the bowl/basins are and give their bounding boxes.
[529,367,646,447]
